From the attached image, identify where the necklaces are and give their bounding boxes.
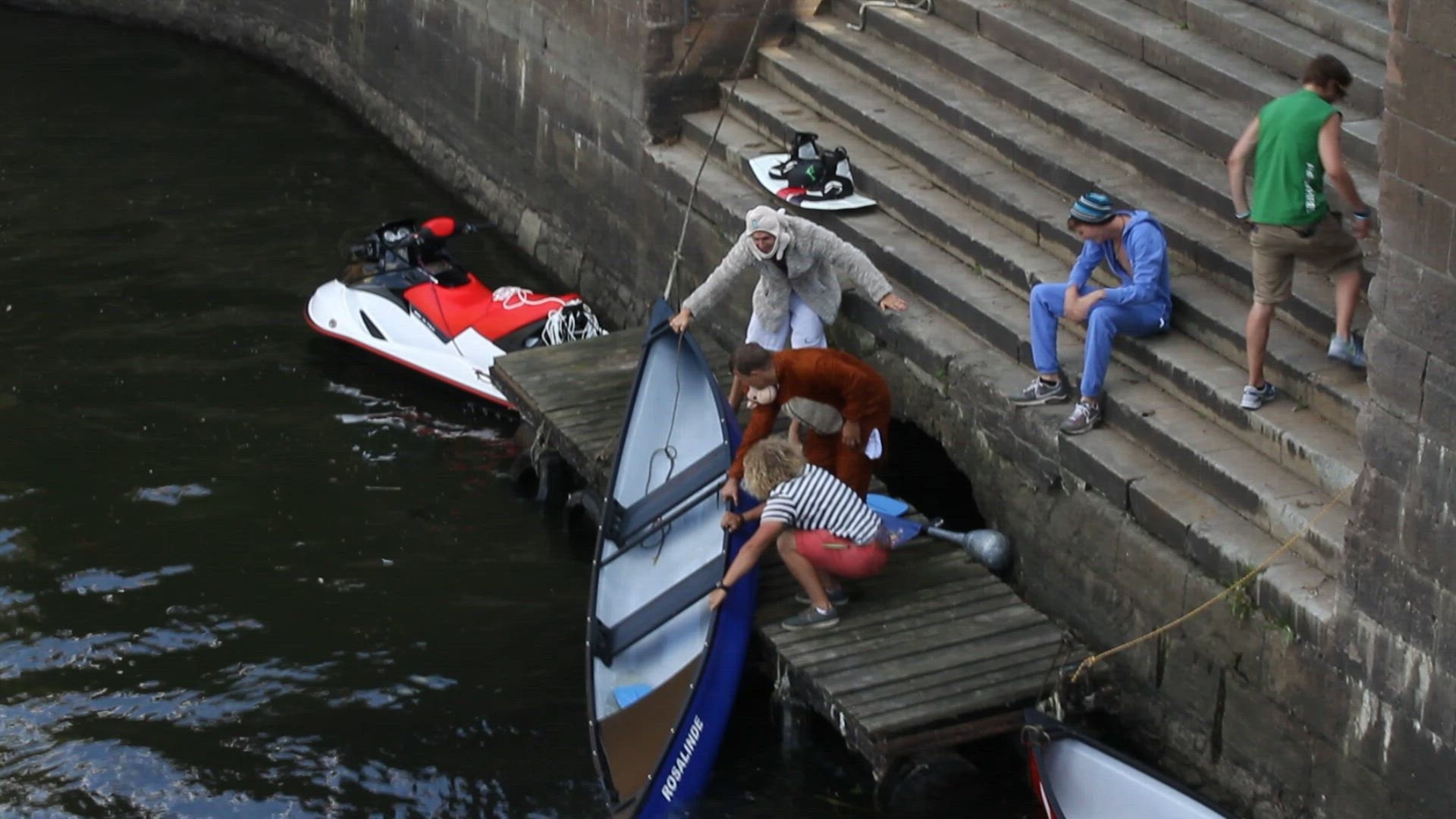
[1114,216,1124,255]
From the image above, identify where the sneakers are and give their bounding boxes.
[1326,336,1368,369]
[1241,381,1274,409]
[781,603,839,630]
[1010,378,1068,406]
[1058,399,1101,435]
[796,585,850,606]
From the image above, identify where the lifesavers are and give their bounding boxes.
[561,488,603,540]
[509,446,564,503]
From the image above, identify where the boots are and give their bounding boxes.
[769,131,819,179]
[803,148,855,202]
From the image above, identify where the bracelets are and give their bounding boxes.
[1235,212,1250,219]
[738,512,744,524]
[716,580,730,592]
[1353,204,1370,218]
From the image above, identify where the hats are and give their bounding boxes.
[1069,192,1115,225]
[745,206,790,261]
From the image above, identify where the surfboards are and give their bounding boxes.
[748,152,879,214]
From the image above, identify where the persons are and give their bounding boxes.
[1006,192,1171,437]
[1229,53,1371,410]
[711,436,892,633]
[669,206,907,350]
[717,340,891,503]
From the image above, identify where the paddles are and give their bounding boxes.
[611,682,653,710]
[864,491,911,518]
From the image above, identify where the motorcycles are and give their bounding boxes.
[303,210,606,421]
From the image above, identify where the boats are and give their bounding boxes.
[1016,705,1230,818]
[567,290,769,818]
